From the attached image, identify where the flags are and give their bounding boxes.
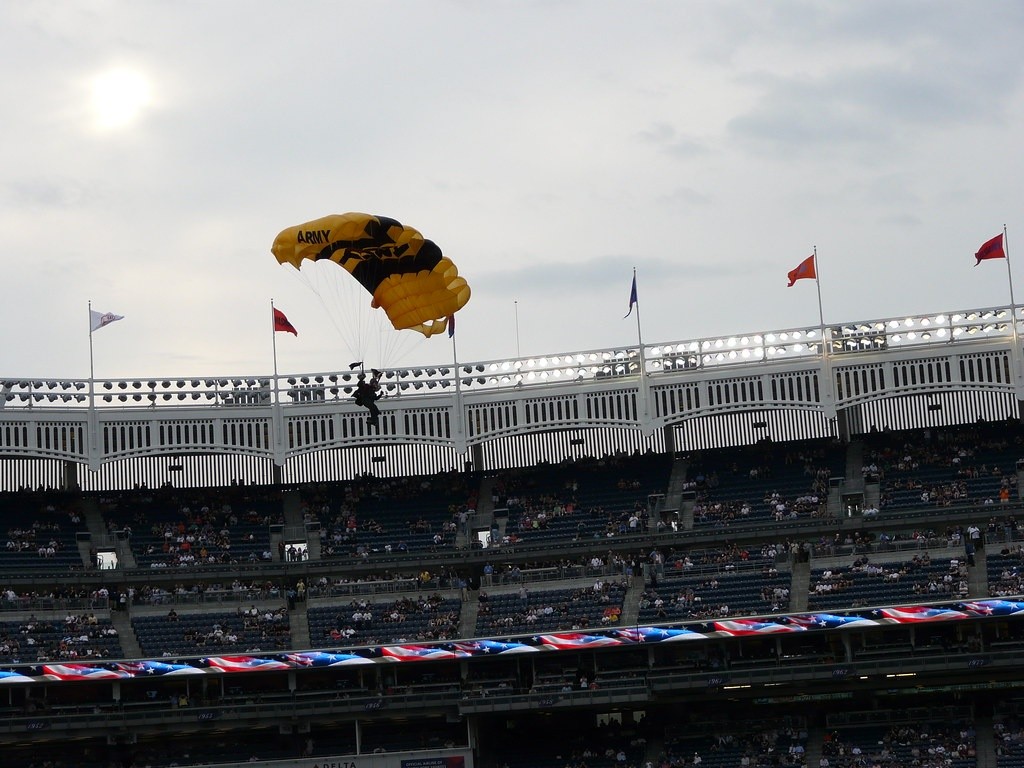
[624,277,637,318]
[91,310,124,331]
[787,255,816,287]
[449,315,455,338]
[273,308,297,337]
[974,234,1005,266]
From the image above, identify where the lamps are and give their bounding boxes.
[0,309,1024,402]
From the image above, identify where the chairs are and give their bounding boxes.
[0,450,1024,768]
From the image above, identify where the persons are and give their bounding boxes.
[351,374,384,426]
[0,416,1024,768]
